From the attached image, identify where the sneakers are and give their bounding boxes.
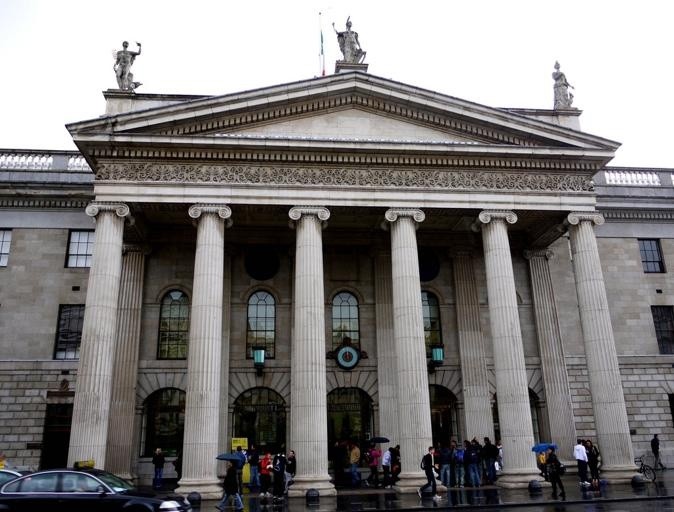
[579,481,590,485]
[433,494,442,500]
[417,489,421,497]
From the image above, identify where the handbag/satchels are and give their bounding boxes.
[559,464,567,476]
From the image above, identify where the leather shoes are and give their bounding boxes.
[592,481,598,484]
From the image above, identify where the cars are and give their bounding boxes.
[0,467,36,491]
[0,467,195,512]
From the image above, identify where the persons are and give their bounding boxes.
[332,16,366,63]
[552,62,574,110]
[152,447,164,488]
[113,41,141,90]
[651,434,666,470]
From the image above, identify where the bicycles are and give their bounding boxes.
[635,454,657,481]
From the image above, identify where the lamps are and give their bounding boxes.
[252,344,267,376]
[428,338,448,374]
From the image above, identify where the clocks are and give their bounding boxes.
[334,343,361,369]
